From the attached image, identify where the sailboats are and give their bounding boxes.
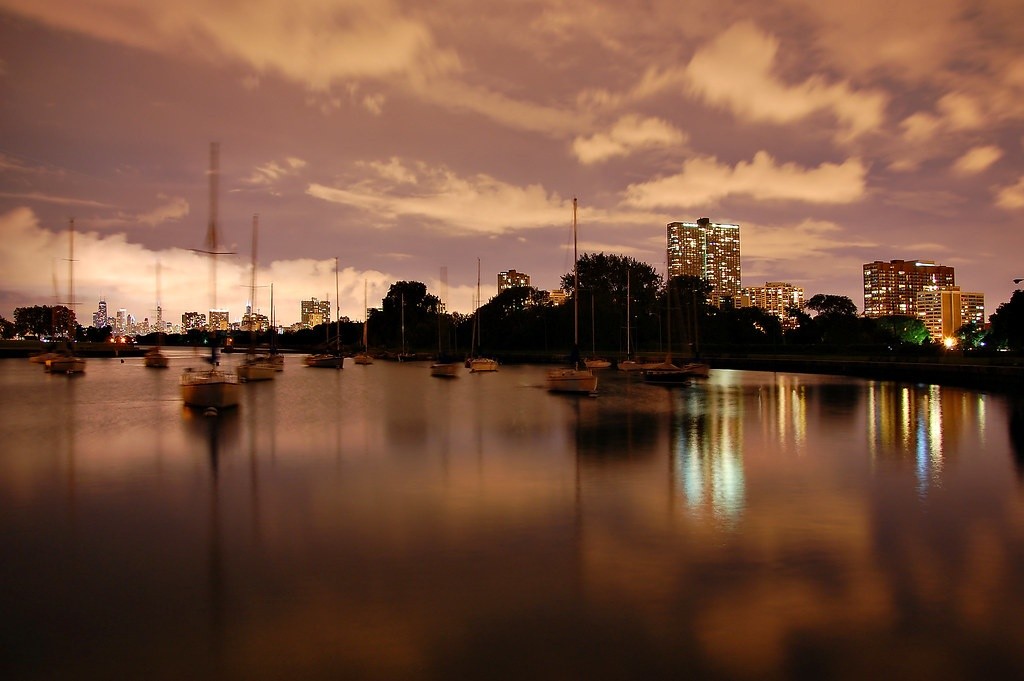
[24,140,711,415]
